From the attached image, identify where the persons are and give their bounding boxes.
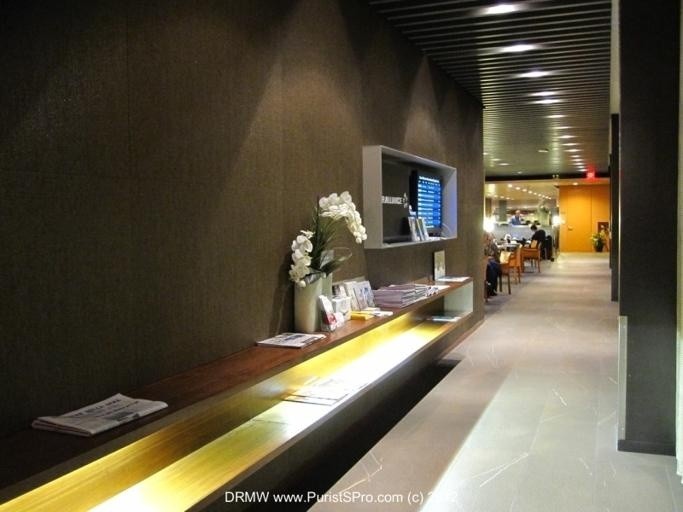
[508,209,524,225]
[531,224,545,250]
[483,232,500,296]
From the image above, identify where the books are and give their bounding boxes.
[318,276,438,332]
[255,332,327,350]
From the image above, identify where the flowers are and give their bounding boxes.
[288,189,367,287]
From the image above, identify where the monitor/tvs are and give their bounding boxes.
[409,169,443,236]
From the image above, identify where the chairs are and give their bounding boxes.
[492,240,542,295]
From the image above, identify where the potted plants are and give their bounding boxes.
[590,232,605,252]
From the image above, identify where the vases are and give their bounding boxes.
[293,277,322,333]
